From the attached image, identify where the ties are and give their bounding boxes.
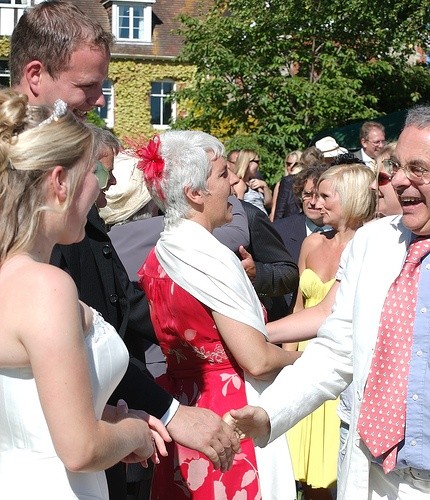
[371,159,378,177]
[356,236,430,474]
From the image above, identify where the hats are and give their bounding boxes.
[315,136,348,158]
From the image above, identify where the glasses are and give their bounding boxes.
[286,161,299,167]
[250,159,259,165]
[377,172,392,186]
[382,159,430,177]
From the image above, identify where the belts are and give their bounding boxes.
[394,465,430,481]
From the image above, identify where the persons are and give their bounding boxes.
[136,130,304,500]
[269,150,302,223]
[98,150,250,354]
[86,122,121,208]
[223,106,430,500]
[0,87,171,500]
[353,122,385,171]
[0,0,242,500]
[315,136,348,165]
[282,162,376,500]
[239,198,299,298]
[271,163,333,319]
[227,148,272,214]
[372,142,403,217]
[274,146,324,220]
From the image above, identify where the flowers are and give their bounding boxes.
[122,131,167,202]
